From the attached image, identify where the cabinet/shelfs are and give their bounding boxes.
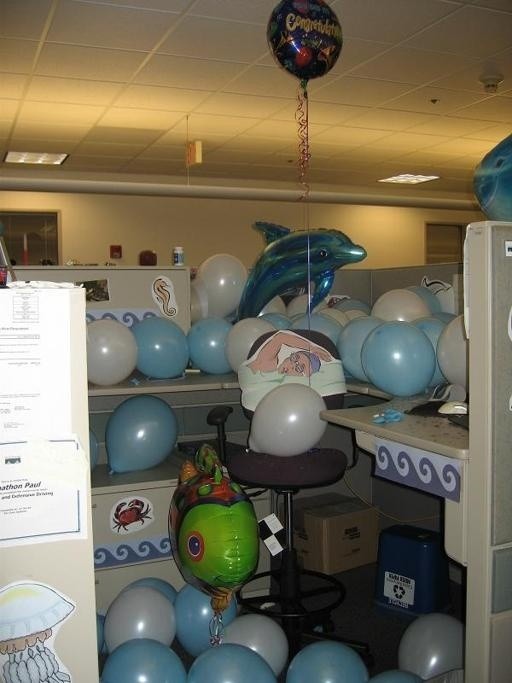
[2,280,101,683]
[93,459,272,628]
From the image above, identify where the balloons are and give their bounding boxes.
[231,222,366,322]
[89,427,99,477]
[105,394,180,477]
[96,577,467,683]
[471,133,512,222]
[247,382,327,458]
[264,0,343,80]
[87,253,468,401]
[167,444,260,615]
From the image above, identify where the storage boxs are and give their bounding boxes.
[282,492,380,576]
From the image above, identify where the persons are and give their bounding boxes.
[238,330,333,410]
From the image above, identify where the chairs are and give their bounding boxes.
[206,329,371,658]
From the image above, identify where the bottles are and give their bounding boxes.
[173,246,184,266]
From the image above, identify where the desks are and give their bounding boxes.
[86,369,466,571]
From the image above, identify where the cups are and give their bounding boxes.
[0,264,8,286]
